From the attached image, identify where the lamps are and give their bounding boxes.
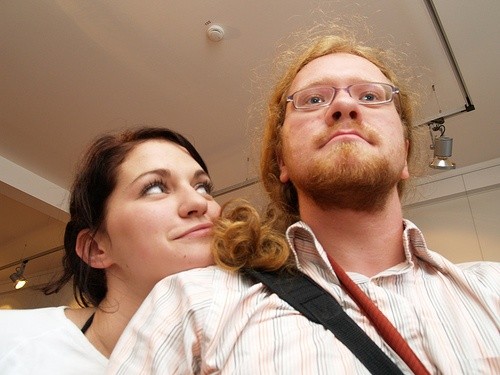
[429,124,456,171]
[10,261,28,291]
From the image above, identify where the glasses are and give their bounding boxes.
[284,83,406,119]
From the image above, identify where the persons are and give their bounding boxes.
[1,128,223,374]
[104,36,499,374]
